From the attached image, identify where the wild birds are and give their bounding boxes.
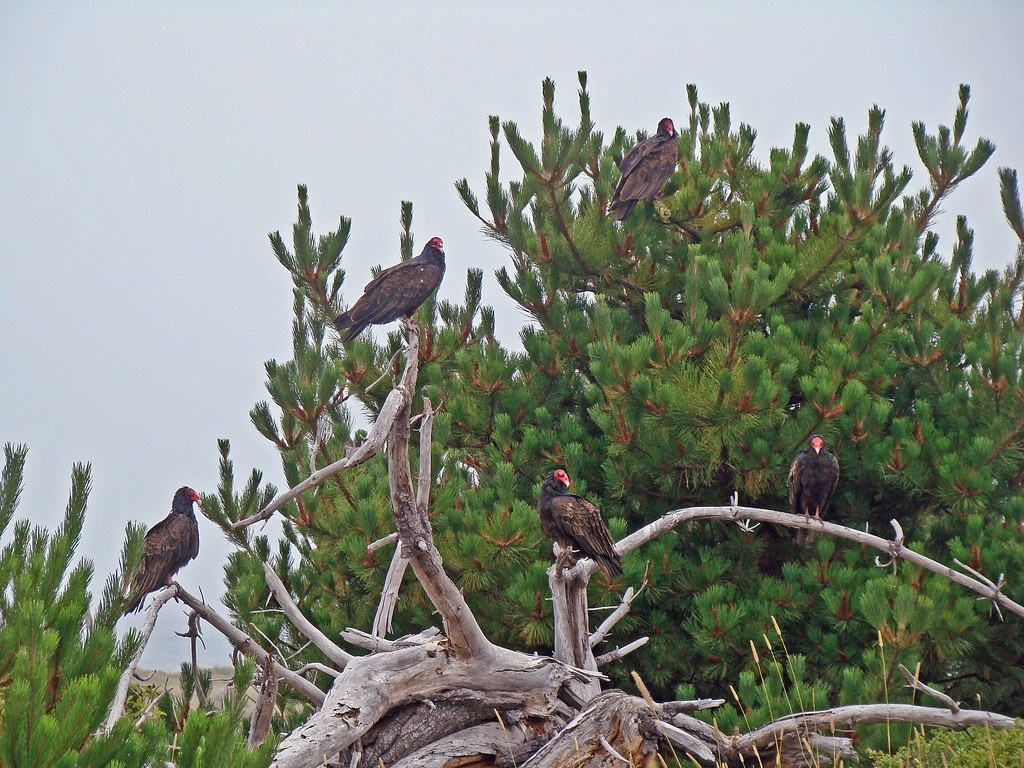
[787,434,839,549]
[607,117,680,222]
[332,235,447,343]
[120,486,204,616]
[539,469,624,584]
